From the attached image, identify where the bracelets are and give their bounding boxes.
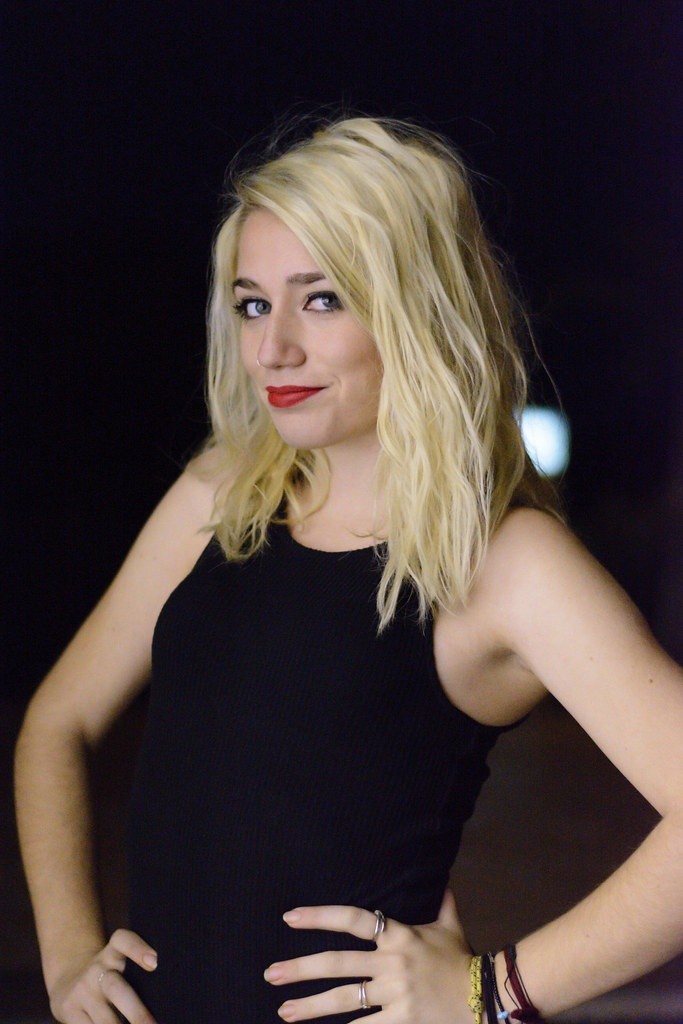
[468,945,546,1024]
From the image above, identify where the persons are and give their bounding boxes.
[14,117,683,1024]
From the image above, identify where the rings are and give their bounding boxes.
[372,910,385,941]
[98,969,123,982]
[358,981,371,1009]
